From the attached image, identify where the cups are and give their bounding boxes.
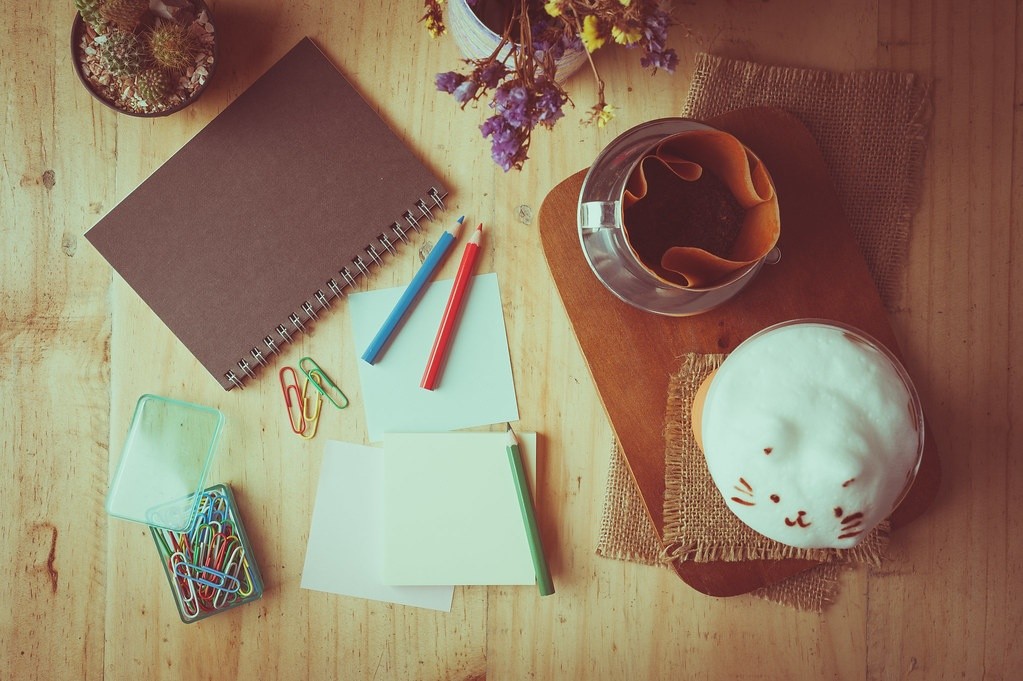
[691,317,926,517]
[581,129,771,293]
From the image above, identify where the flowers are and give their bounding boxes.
[416,1,698,175]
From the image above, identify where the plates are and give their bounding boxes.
[576,117,779,317]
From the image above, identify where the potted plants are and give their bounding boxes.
[70,1,220,120]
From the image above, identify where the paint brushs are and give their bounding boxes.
[361,216,484,392]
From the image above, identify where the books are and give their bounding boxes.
[85,35,450,392]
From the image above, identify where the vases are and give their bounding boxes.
[445,1,593,89]
